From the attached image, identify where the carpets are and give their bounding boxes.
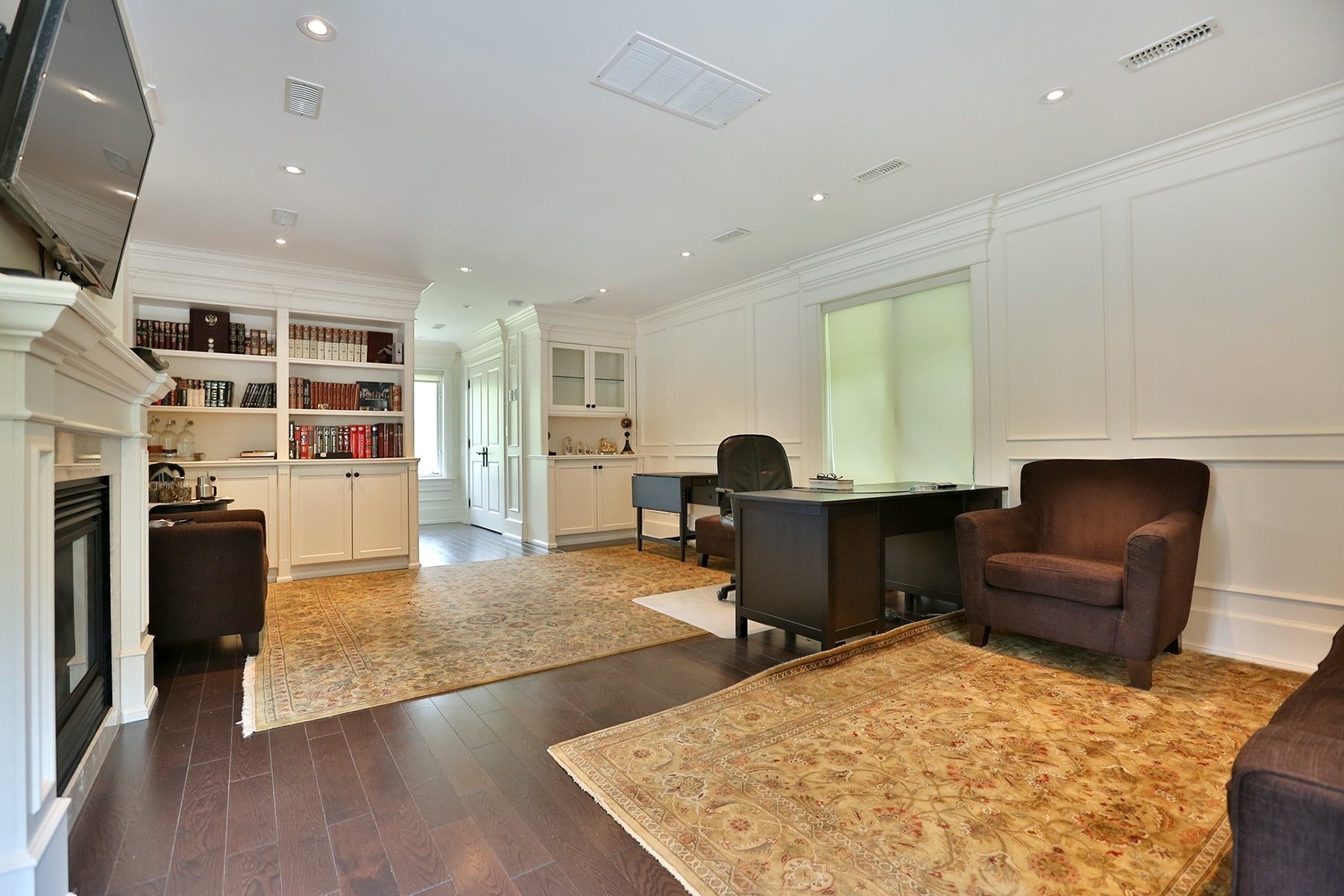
[632,581,777,639]
[547,607,1311,896]
[239,542,763,738]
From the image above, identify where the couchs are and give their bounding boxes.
[954,458,1210,689]
[149,509,268,657]
[1225,625,1344,896]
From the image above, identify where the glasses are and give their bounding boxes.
[817,473,843,480]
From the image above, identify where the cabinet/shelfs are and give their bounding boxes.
[283,308,420,579]
[547,342,631,416]
[129,295,282,583]
[464,354,504,534]
[549,459,646,546]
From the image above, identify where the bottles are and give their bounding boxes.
[148,415,194,461]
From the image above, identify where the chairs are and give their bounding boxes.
[715,434,792,601]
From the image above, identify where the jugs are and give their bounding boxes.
[196,476,217,500]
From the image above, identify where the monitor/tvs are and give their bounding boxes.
[0,0,156,298]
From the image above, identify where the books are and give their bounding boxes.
[288,323,403,365]
[151,377,277,409]
[137,308,277,358]
[289,377,402,411]
[809,478,854,491]
[228,450,276,460]
[289,420,404,459]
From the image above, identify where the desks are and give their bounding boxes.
[631,472,719,561]
[727,481,1008,654]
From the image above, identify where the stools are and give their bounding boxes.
[695,514,734,568]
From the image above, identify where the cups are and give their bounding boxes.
[151,481,193,503]
[194,453,205,460]
